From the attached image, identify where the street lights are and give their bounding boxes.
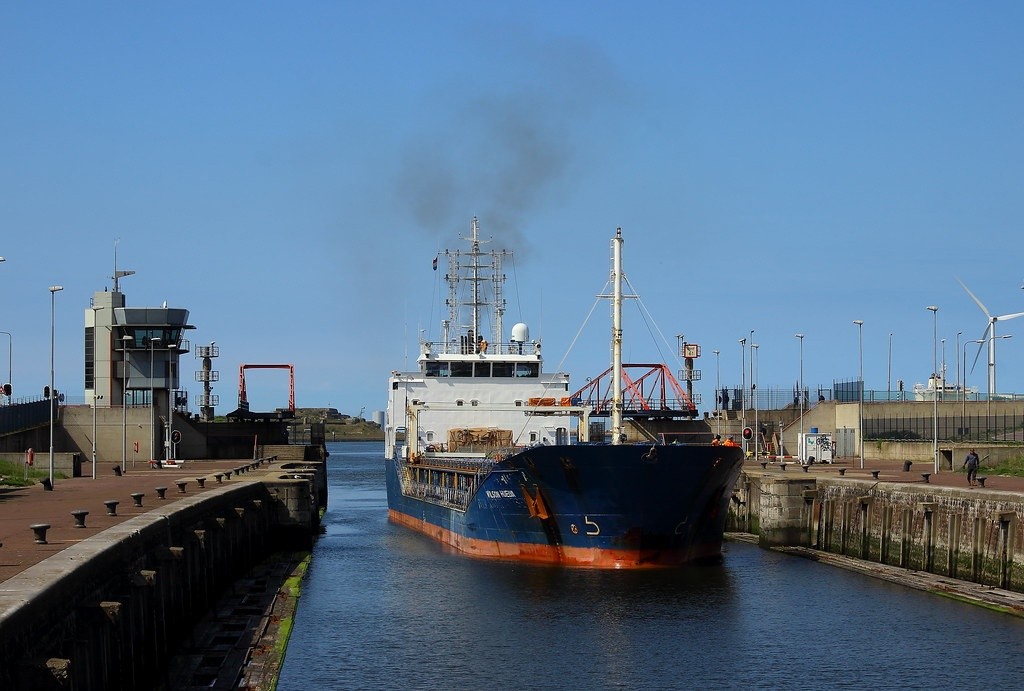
[794,333,805,466]
[888,333,895,401]
[853,319,865,470]
[926,305,942,475]
[121,335,133,474]
[712,350,722,443]
[749,329,755,409]
[986,335,1013,435]
[149,337,160,470]
[91,304,105,481]
[47,285,64,487]
[167,344,177,460]
[750,343,759,462]
[738,338,747,452]
[956,331,961,402]
[962,339,988,438]
[941,337,946,400]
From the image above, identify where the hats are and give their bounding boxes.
[970,447,975,450]
[727,435,733,438]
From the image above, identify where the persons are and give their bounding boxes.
[723,435,742,448]
[712,434,721,446]
[962,449,979,486]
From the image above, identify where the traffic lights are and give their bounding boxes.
[3,383,12,396]
[44,386,50,399]
[742,427,753,441]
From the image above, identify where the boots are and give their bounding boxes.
[968,481,971,485]
[972,481,978,485]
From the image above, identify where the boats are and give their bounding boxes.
[383,212,754,573]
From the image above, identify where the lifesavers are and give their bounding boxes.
[495,453,501,463]
